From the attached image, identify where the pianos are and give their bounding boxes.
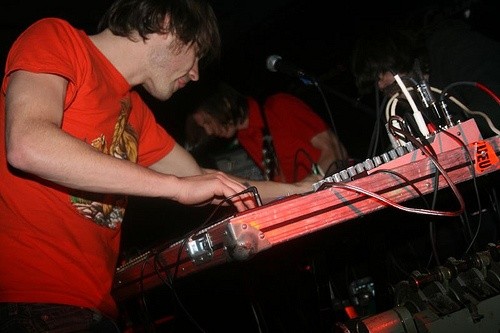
[113,117,500,333]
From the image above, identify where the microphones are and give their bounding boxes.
[266,55,318,86]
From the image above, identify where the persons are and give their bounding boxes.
[183,76,354,333]
[0,0,324,333]
[362,36,456,144]
